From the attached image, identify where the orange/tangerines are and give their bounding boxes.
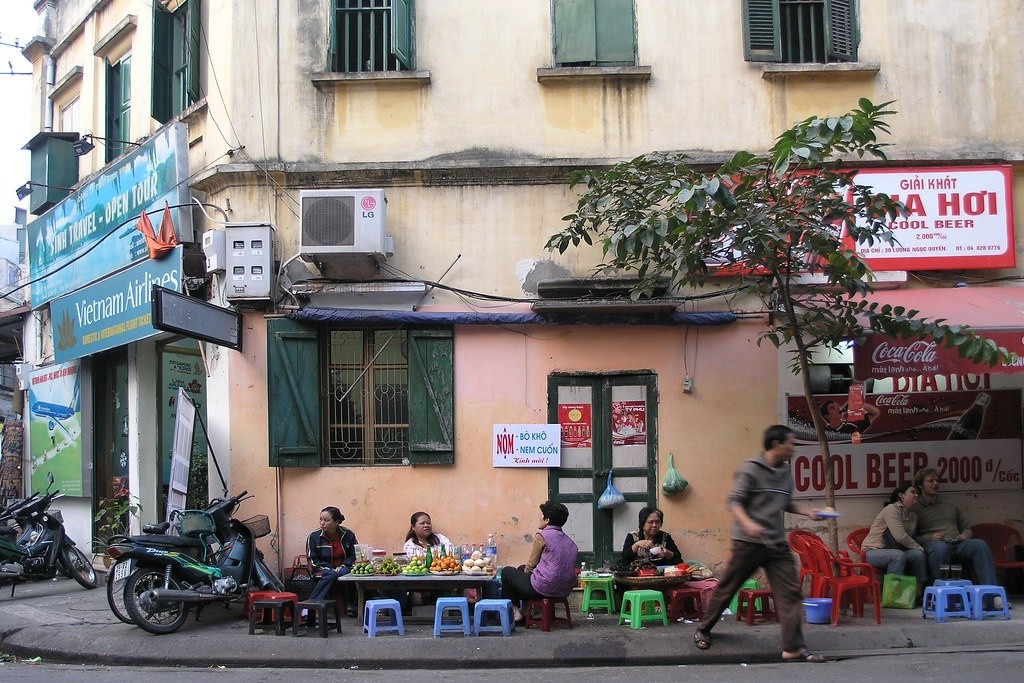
[428,556,462,572]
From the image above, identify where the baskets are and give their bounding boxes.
[242,515,271,538]
[47,510,63,524]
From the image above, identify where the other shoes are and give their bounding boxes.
[346,606,357,616]
[301,614,308,621]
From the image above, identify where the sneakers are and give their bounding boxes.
[994,597,1011,610]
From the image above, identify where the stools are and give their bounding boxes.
[580,576,780,629]
[433,597,515,637]
[921,579,1011,621]
[247,591,342,638]
[363,599,405,637]
[525,598,572,633]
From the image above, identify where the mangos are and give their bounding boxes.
[351,563,375,573]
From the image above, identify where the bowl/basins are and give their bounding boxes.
[650,546,661,555]
[801,597,832,624]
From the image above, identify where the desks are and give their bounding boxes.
[615,574,691,586]
[338,566,500,624]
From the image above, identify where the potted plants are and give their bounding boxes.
[91,491,144,568]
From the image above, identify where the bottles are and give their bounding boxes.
[440,544,447,560]
[946,391,991,440]
[449,543,454,560]
[371,550,387,572]
[470,543,477,554]
[432,545,439,562]
[426,544,433,568]
[461,543,471,570]
[580,562,588,577]
[392,552,411,565]
[486,533,498,574]
[477,543,487,558]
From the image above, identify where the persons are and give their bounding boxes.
[694,425,826,662]
[910,468,1012,610]
[860,484,932,610]
[625,413,635,428]
[403,512,454,564]
[620,506,683,566]
[501,501,578,627]
[300,507,359,620]
[820,400,879,434]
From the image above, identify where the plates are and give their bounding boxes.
[462,570,487,575]
[598,573,612,578]
[353,573,427,576]
[430,570,458,575]
[815,512,840,518]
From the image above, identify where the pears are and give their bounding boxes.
[462,551,491,573]
[402,557,428,573]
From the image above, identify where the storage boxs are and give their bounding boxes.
[686,578,719,613]
[285,579,315,601]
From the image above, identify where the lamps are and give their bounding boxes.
[72,129,142,156]
[16,181,76,200]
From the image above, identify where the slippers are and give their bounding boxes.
[787,651,828,662]
[694,628,712,649]
[514,619,525,626]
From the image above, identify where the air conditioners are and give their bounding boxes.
[299,188,394,262]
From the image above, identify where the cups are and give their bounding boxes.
[940,564,950,580]
[603,563,610,572]
[951,565,961,581]
[354,543,369,564]
[367,547,374,562]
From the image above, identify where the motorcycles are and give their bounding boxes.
[0,472,99,592]
[104,489,285,635]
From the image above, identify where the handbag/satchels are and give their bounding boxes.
[598,471,625,509]
[662,454,688,496]
[284,554,320,579]
[882,574,917,608]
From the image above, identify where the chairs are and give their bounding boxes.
[973,524,1024,569]
[787,527,883,627]
